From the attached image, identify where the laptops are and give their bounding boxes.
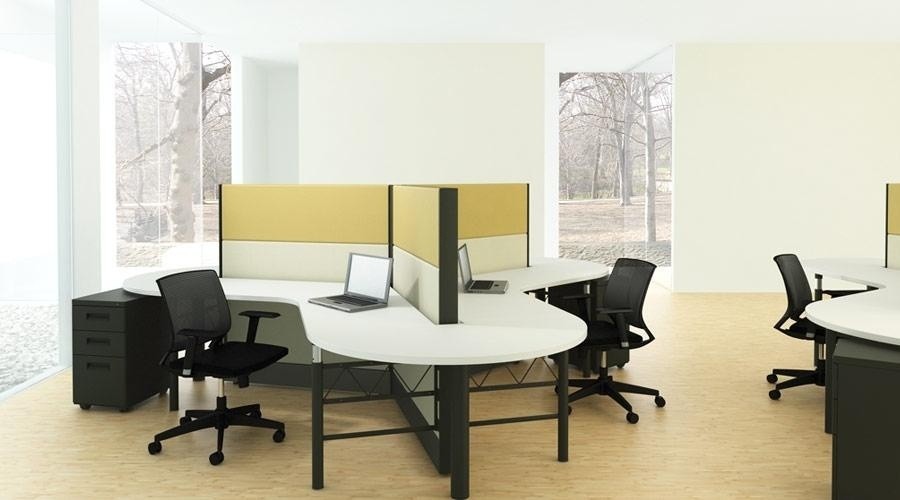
[457,243,509,294]
[308,252,394,312]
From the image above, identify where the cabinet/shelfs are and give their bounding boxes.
[832,338,900,500]
[72,288,170,411]
[548,274,629,375]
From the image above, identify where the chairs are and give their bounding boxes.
[767,254,826,399]
[555,258,666,424]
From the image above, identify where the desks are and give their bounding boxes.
[805,257,900,433]
